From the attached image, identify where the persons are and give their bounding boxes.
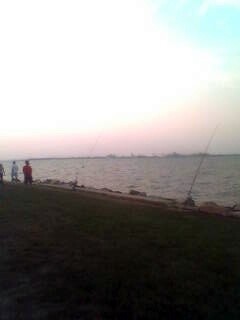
[22,160,33,186]
[11,161,19,185]
[0,163,6,183]
